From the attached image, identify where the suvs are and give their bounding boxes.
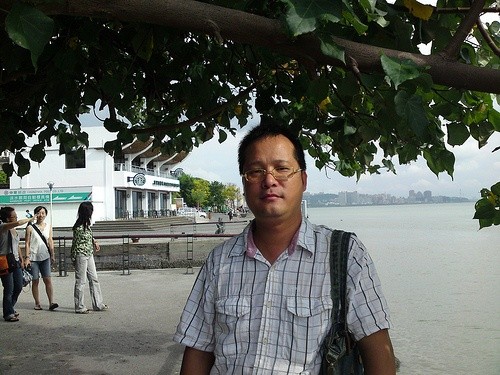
[178,207,207,218]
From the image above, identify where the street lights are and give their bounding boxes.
[46,180,55,227]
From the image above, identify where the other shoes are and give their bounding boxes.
[5,311,19,321]
[34,305,42,310]
[76,308,92,314]
[49,303,58,310]
[93,304,108,310]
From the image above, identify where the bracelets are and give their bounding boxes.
[24,256,30,259]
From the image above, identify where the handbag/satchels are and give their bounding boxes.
[0,253,16,277]
[23,269,33,287]
[320,230,365,375]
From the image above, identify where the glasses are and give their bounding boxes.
[241,168,302,183]
[10,215,17,219]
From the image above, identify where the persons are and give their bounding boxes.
[226,208,241,221]
[71,201,109,314]
[173,123,397,375]
[215,217,226,235]
[24,205,59,310]
[24,210,33,231]
[0,206,38,322]
